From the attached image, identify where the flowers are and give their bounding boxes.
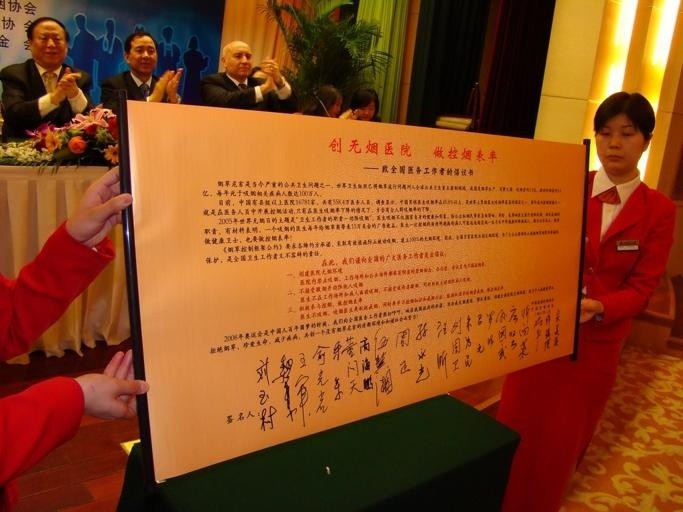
[0,104,119,174]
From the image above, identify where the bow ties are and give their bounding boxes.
[594,185,622,205]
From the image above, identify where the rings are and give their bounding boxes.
[270,67,273,71]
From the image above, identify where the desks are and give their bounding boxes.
[113,396,525,508]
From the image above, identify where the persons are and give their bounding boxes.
[99,30,186,122]
[0,166,151,511]
[0,17,96,146]
[494,91,676,512]
[349,87,381,122]
[292,85,341,118]
[196,40,299,115]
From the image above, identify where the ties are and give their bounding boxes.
[42,72,57,95]
[238,83,246,89]
[139,83,150,101]
